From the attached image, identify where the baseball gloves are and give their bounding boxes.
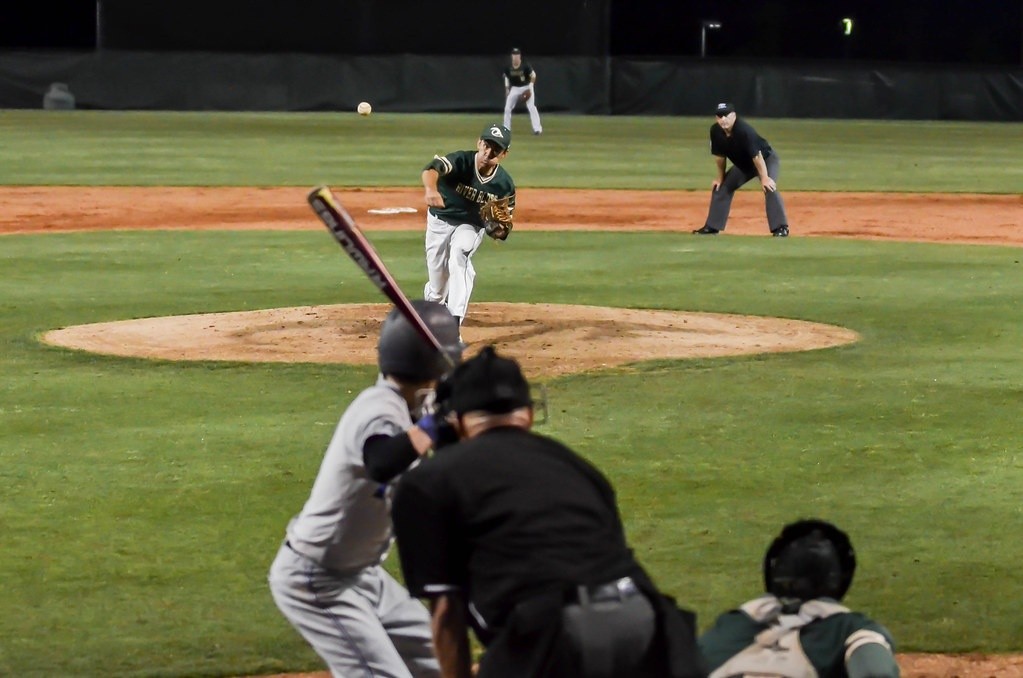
[518,89,531,103]
[478,196,513,241]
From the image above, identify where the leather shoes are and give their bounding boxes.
[773,227,789,236]
[692,225,719,234]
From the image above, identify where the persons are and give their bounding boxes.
[269,300,461,678]
[423,123,517,343]
[389,347,668,678]
[692,103,790,237]
[503,47,543,133]
[699,518,902,678]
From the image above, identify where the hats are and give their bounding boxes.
[511,48,521,53]
[480,122,511,150]
[715,102,736,114]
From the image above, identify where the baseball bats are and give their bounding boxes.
[304,186,457,371]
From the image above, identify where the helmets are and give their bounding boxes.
[763,520,857,601]
[434,346,530,414]
[377,300,459,379]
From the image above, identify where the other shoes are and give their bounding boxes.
[535,131,540,135]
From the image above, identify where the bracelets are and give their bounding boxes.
[417,412,458,449]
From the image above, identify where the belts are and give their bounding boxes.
[492,577,642,624]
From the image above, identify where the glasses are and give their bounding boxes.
[717,113,729,118]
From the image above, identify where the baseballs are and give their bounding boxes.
[357,101,373,115]
